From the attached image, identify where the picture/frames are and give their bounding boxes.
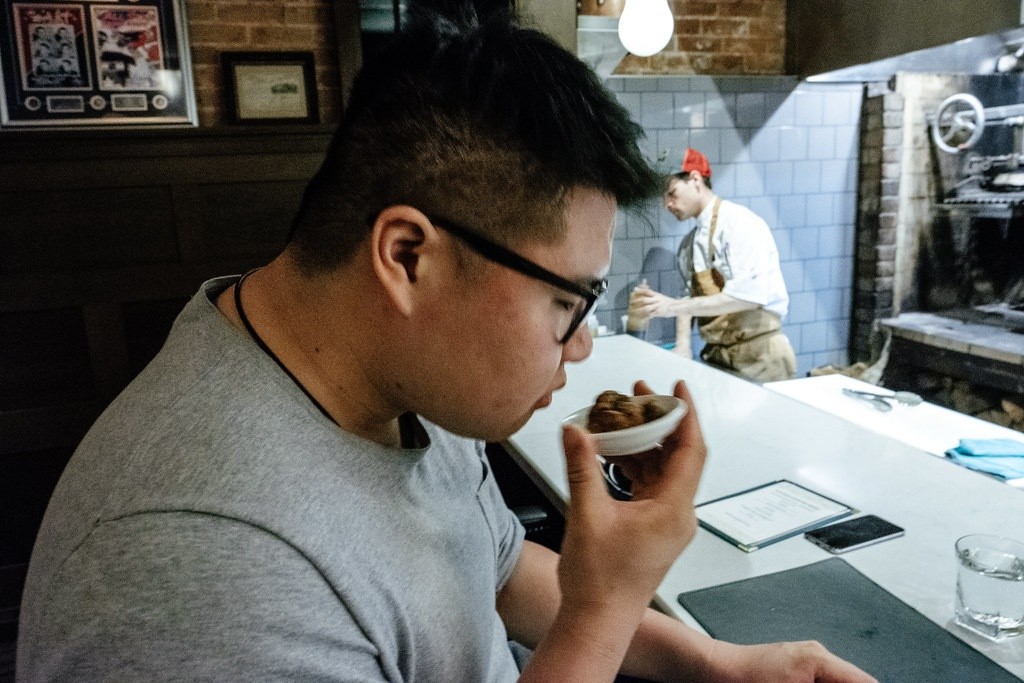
[0,0,201,131]
[219,49,322,128]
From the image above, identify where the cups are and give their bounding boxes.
[954,533,1023,644]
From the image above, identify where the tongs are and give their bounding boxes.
[842,387,924,411]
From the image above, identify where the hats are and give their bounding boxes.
[651,148,712,178]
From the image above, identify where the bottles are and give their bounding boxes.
[626,279,651,341]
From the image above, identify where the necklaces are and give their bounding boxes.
[234,267,340,427]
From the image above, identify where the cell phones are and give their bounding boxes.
[805,514,905,555]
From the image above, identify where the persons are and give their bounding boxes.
[31,26,77,75]
[632,147,796,384]
[14,24,879,683]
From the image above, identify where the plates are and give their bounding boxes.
[562,395,688,456]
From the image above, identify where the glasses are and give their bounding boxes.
[430,213,607,344]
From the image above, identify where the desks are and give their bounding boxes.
[502,329,1024,683]
